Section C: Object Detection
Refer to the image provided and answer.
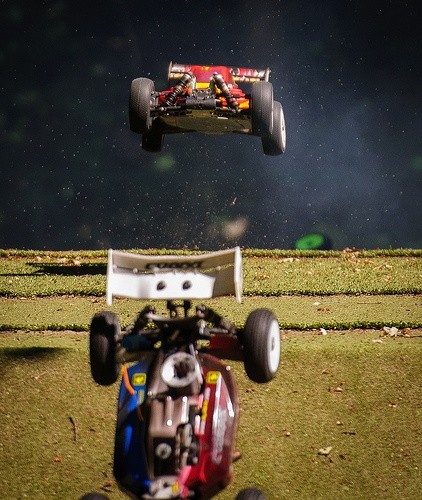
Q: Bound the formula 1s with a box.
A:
[78,244,284,500]
[128,61,289,156]
